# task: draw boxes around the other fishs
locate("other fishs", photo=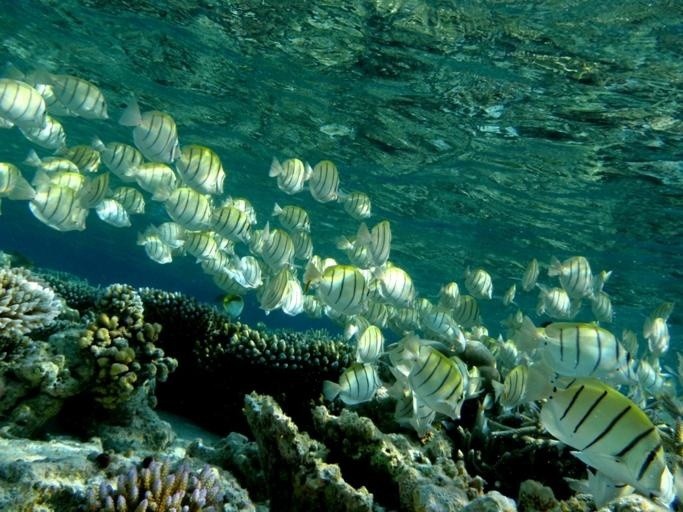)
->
[0,73,683,512]
